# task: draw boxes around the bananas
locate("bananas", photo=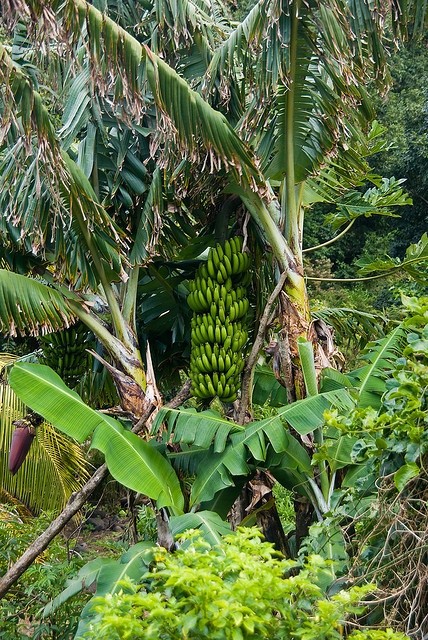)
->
[186,236,250,408]
[36,324,91,379]
